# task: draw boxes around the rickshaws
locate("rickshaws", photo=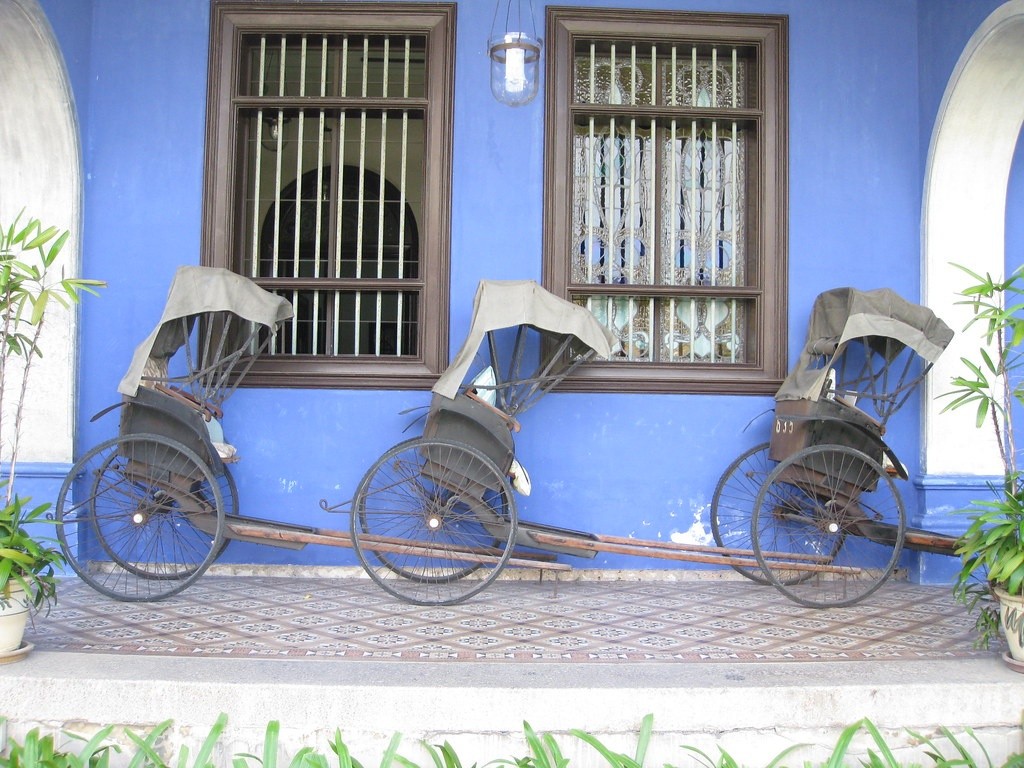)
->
[316,276,864,610]
[55,264,575,604]
[711,285,997,611]
